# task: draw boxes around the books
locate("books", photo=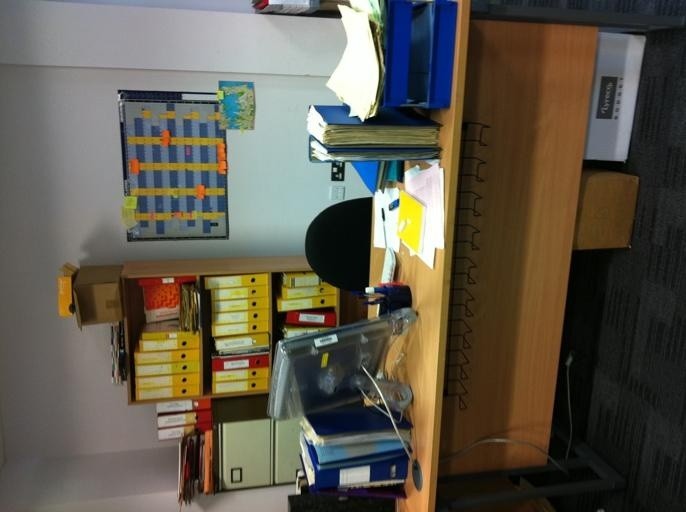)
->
[301,409,413,491]
[308,134,440,160]
[395,188,426,255]
[306,105,445,148]
